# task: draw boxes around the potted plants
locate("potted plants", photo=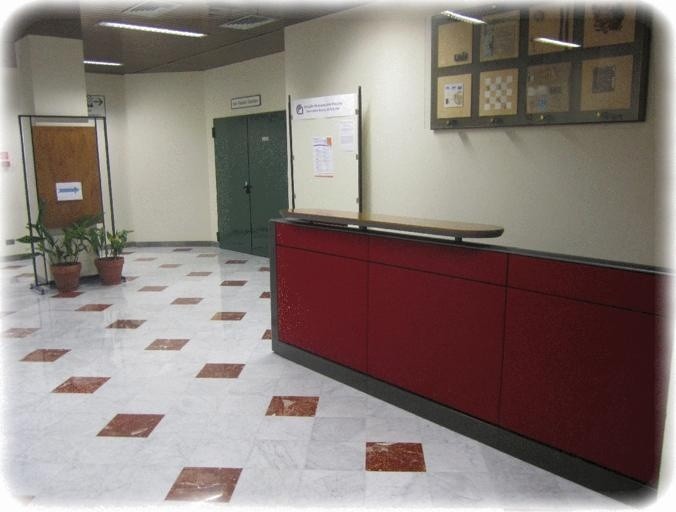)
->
[16,200,105,291]
[63,220,134,284]
